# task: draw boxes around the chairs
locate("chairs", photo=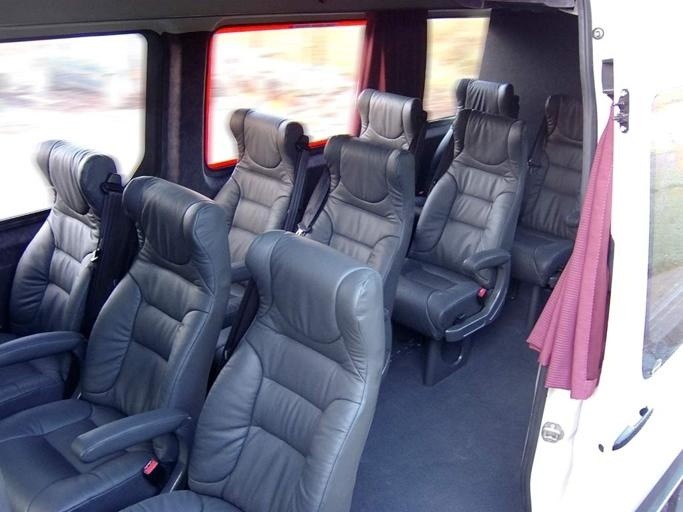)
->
[71,230,385,511]
[393,107,528,386]
[212,109,311,367]
[1,175,231,511]
[0,140,140,420]
[510,94,583,335]
[296,135,416,317]
[356,89,428,185]
[415,78,520,218]
